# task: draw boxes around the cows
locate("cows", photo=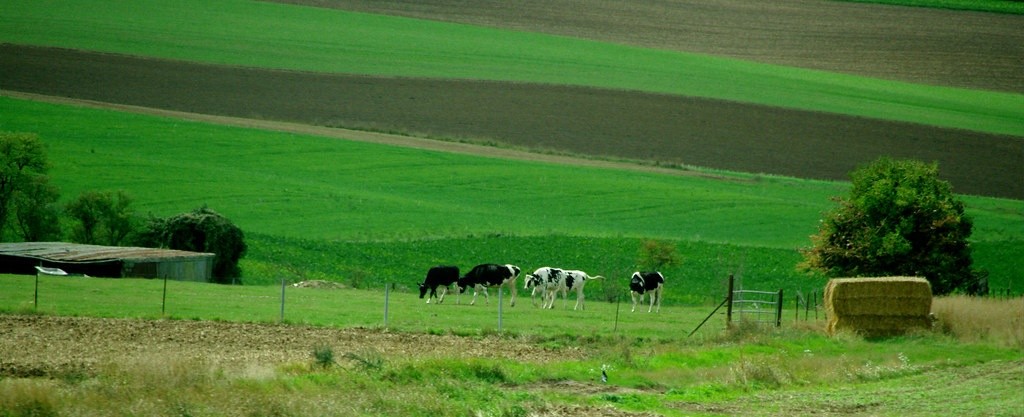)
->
[630,272,664,312]
[417,264,520,306]
[524,266,605,311]
